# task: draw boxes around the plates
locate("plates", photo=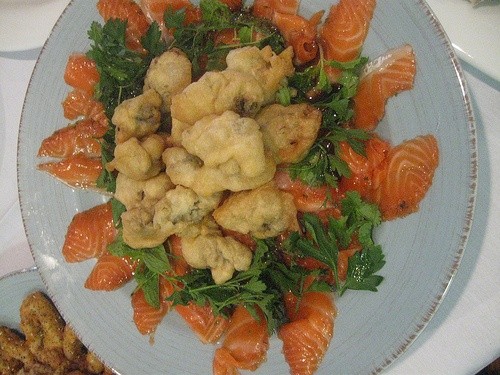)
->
[17,0,479,375]
